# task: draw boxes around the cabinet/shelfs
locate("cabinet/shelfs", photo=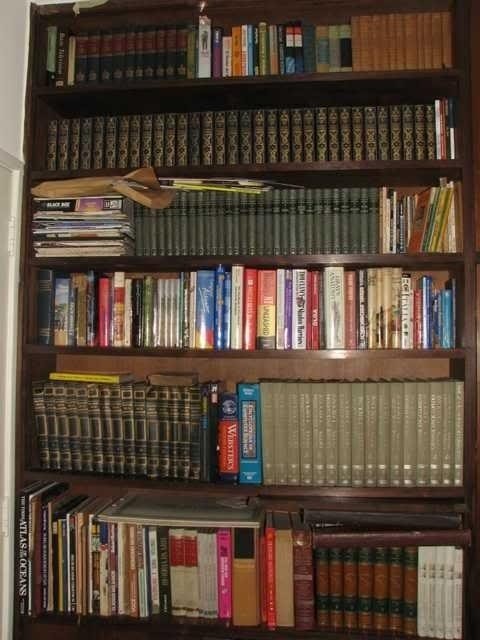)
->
[11,5,480,637]
